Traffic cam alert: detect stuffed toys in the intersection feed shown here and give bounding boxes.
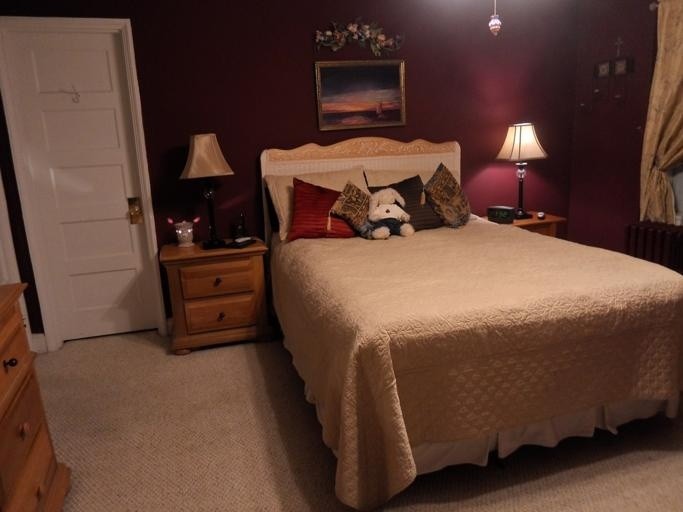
[365,186,414,239]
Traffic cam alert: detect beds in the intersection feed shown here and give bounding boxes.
[260,136,683,512]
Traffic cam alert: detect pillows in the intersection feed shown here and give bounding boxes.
[424,162,471,228]
[368,174,445,232]
[263,166,372,241]
[286,178,354,243]
[362,169,458,189]
[328,180,372,231]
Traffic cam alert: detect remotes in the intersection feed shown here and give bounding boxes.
[234,236,251,243]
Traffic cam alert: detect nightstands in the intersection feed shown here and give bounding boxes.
[480,210,566,239]
[158,237,268,355]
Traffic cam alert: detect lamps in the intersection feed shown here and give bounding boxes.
[495,122,549,219]
[178,133,234,249]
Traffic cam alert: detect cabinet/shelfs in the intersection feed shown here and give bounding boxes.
[0,281,71,512]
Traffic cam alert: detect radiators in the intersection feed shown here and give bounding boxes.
[624,222,682,273]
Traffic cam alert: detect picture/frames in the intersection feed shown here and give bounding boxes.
[314,58,405,131]
